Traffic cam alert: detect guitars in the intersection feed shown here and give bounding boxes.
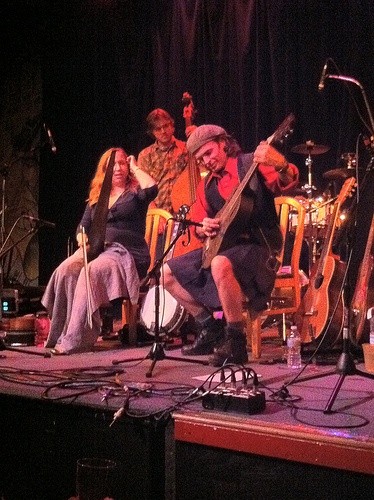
[290,174,359,352]
[199,112,299,270]
[347,212,374,351]
[140,204,190,339]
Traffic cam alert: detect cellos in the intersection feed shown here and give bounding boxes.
[167,90,213,259]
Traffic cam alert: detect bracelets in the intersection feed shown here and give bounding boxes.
[275,162,289,175]
[133,167,140,174]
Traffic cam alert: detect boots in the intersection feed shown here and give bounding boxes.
[98,306,118,340]
[181,319,225,355]
[209,330,248,365]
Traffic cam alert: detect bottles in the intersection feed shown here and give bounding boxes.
[287,326,301,370]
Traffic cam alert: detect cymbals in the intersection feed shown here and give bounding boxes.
[321,168,362,180]
[290,143,330,155]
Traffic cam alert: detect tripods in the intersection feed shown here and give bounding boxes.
[112,227,210,377]
[287,156,374,414]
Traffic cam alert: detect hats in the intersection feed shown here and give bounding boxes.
[187,123,225,153]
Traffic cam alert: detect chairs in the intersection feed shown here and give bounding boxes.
[241,196,305,360]
[112,209,176,344]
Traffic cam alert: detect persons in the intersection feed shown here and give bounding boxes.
[159,125,299,366]
[138,109,207,343]
[41,148,158,354]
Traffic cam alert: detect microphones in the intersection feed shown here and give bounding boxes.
[319,63,327,90]
[47,128,56,151]
[171,217,203,227]
[23,215,56,227]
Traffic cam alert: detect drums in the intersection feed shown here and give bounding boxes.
[291,201,343,240]
